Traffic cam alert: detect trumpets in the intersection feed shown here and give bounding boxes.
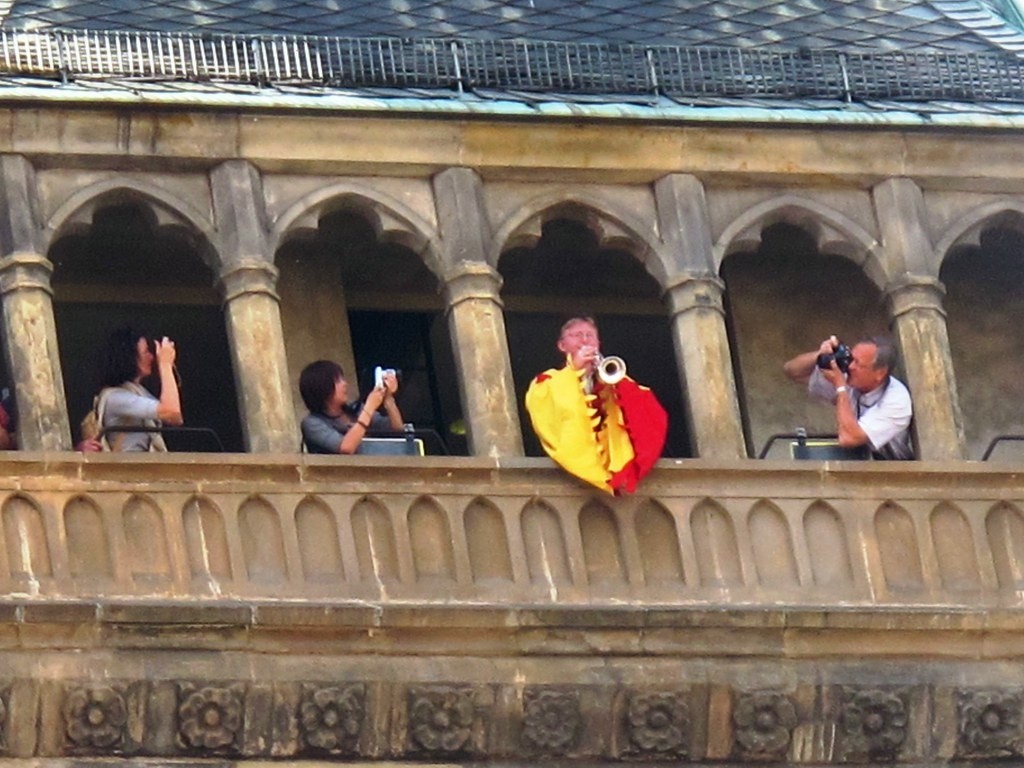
[591,351,627,385]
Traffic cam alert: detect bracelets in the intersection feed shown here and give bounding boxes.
[834,385,850,395]
[355,415,373,435]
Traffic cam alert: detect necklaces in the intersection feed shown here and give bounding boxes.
[321,409,344,419]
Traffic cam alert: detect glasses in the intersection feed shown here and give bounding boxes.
[560,331,599,343]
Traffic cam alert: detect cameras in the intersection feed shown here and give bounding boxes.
[374,367,396,389]
[817,342,852,374]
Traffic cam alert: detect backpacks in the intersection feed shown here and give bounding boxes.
[76,389,134,453]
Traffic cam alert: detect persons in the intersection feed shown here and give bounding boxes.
[783,335,915,461]
[95,325,184,454]
[298,358,404,455]
[525,316,670,498]
[67,405,104,454]
